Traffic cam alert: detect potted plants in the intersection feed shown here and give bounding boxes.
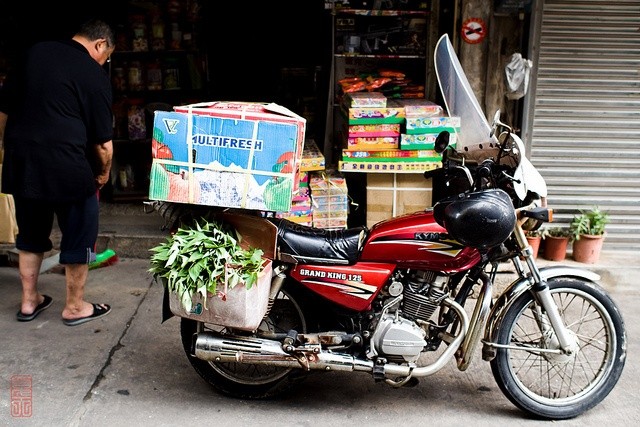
[521,230,542,257]
[571,206,608,264]
[543,224,572,259]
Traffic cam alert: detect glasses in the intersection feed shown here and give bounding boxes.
[105,52,112,62]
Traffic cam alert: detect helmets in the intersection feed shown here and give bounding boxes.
[432,190,516,250]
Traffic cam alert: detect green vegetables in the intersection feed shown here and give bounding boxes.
[145,217,267,315]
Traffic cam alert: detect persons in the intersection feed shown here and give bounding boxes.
[1,17,117,327]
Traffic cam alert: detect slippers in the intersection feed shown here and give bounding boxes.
[65,301,111,326]
[15,294,53,321]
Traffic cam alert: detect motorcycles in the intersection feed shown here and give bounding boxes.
[143,32,629,421]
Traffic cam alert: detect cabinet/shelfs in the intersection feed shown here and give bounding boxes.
[331,1,431,108]
[105,1,200,199]
